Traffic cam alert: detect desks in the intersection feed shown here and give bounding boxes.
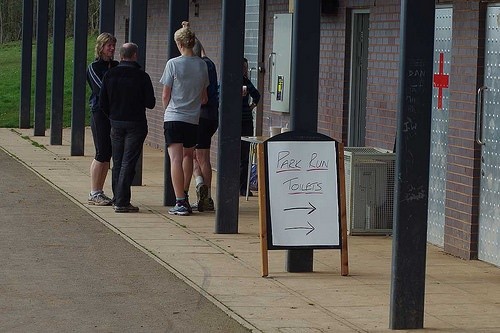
[241,135,270,201]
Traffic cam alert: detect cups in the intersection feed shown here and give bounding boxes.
[243,85,247,96]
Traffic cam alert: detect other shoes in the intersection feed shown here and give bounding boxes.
[241,190,252,196]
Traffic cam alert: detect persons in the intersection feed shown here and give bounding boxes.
[159,28,210,215]
[182,21,219,211]
[99,42,155,212]
[240,58,261,196]
[87,33,119,204]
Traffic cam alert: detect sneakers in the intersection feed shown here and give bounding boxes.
[196,183,209,212]
[168,204,189,214]
[192,198,214,210]
[115,204,139,212]
[88,191,112,205]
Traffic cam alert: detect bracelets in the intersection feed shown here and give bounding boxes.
[252,102,256,106]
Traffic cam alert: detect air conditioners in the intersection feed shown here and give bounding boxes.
[344,158,388,236]
